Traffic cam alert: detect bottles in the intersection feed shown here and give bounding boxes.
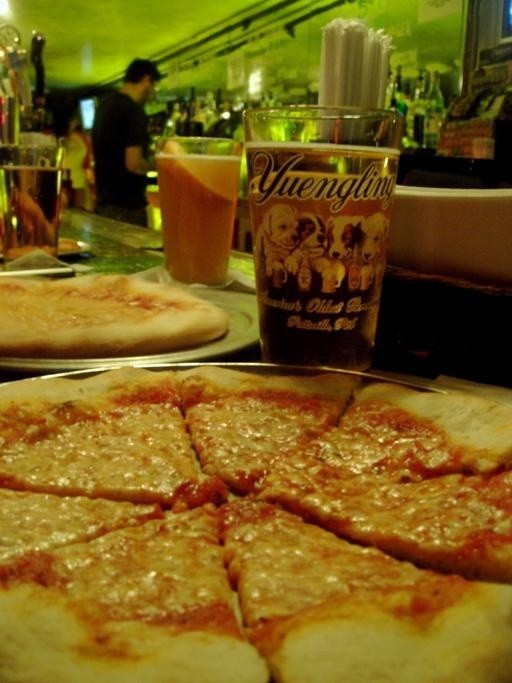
[392,61,445,147]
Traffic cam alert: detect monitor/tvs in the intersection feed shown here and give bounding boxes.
[78,96,98,133]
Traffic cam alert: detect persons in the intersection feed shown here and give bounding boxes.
[91,58,160,228]
[59,110,87,208]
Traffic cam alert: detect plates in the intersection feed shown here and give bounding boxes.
[0,361,453,402]
[4,271,258,366]
[0,236,96,260]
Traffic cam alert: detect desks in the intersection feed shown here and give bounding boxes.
[1,208,512,384]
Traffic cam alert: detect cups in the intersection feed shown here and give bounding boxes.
[0,139,64,262]
[155,134,244,284]
[242,103,404,366]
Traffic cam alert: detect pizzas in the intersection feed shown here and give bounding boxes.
[0,275,227,358]
[0,366,511,682]
